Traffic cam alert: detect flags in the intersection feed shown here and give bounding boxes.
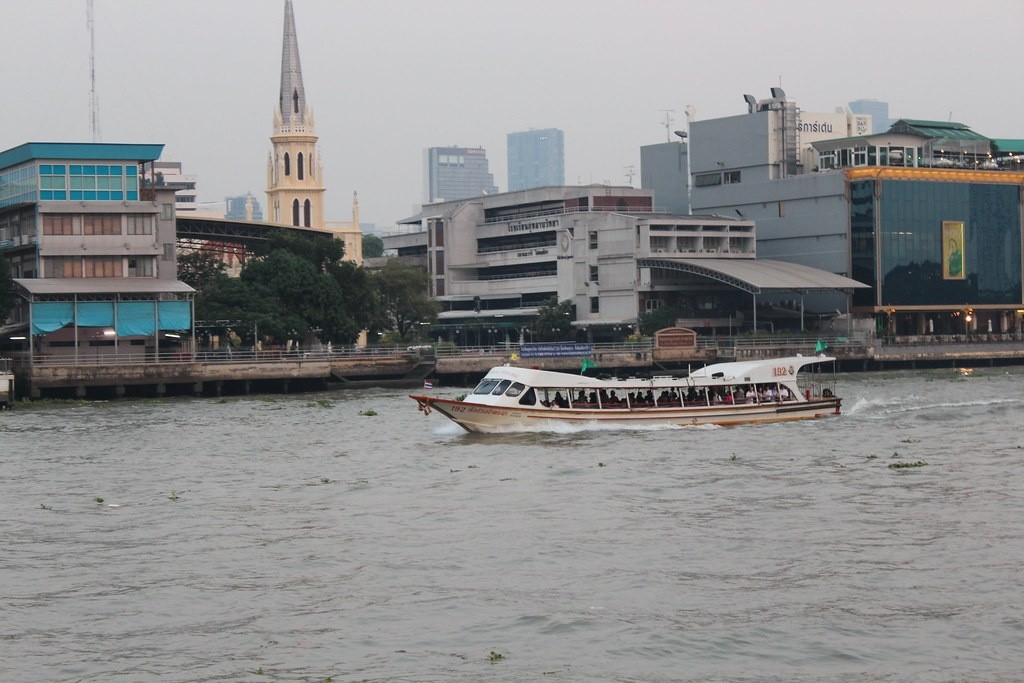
[424,379,433,389]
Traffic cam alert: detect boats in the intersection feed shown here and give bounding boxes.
[409,354,842,434]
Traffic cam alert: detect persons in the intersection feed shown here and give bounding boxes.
[578,387,788,403]
[551,392,565,407]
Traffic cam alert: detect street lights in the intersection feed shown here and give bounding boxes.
[965,303,973,344]
[887,303,896,345]
